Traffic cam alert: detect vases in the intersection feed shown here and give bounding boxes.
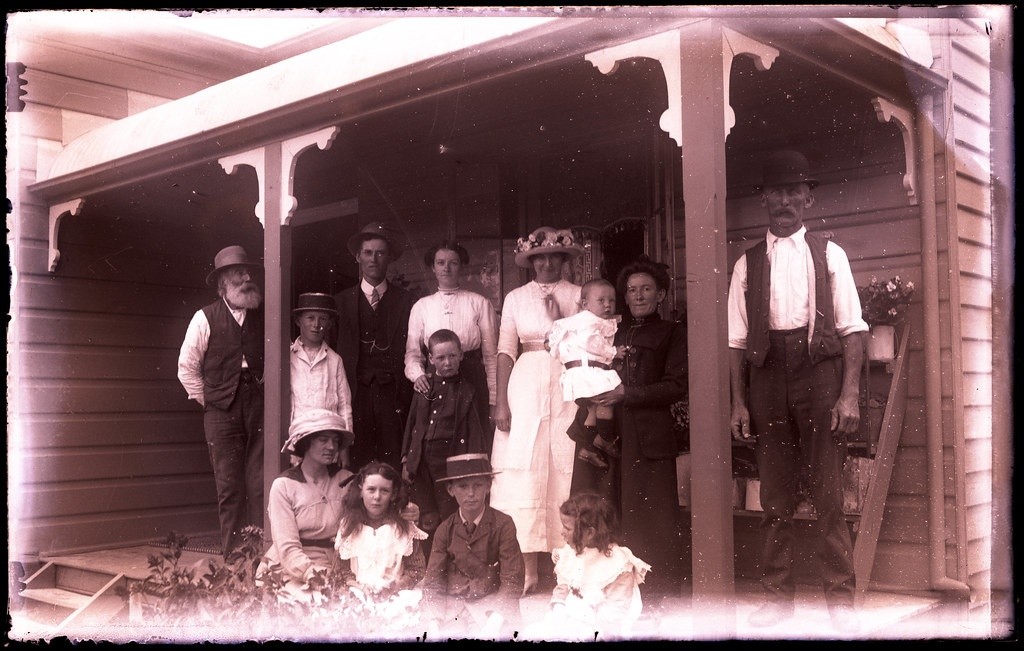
[867,326,898,364]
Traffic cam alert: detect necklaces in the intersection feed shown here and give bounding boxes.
[434,290,458,309]
[535,280,557,298]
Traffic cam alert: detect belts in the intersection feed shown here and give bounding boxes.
[301,537,336,549]
[565,359,609,370]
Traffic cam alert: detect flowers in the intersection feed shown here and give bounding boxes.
[514,229,574,255]
[857,274,916,338]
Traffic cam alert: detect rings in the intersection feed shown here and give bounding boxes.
[600,399,607,404]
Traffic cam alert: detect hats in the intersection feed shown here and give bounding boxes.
[280,410,354,458]
[291,293,338,318]
[346,222,404,263]
[435,454,502,482]
[514,227,587,268]
[751,150,819,190]
[205,246,263,288]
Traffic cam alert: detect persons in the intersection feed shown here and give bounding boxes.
[417,450,525,631]
[728,150,870,608]
[548,277,631,471]
[254,408,421,600]
[399,329,489,569]
[405,240,496,456]
[570,254,689,604]
[289,291,356,475]
[177,243,264,557]
[546,492,652,630]
[333,460,430,616]
[489,225,590,593]
[322,221,420,478]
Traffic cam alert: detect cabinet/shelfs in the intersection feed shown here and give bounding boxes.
[733,323,911,605]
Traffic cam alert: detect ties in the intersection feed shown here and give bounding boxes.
[371,288,381,311]
[466,521,476,537]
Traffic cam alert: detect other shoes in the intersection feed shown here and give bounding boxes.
[578,448,609,469]
[594,435,622,459]
